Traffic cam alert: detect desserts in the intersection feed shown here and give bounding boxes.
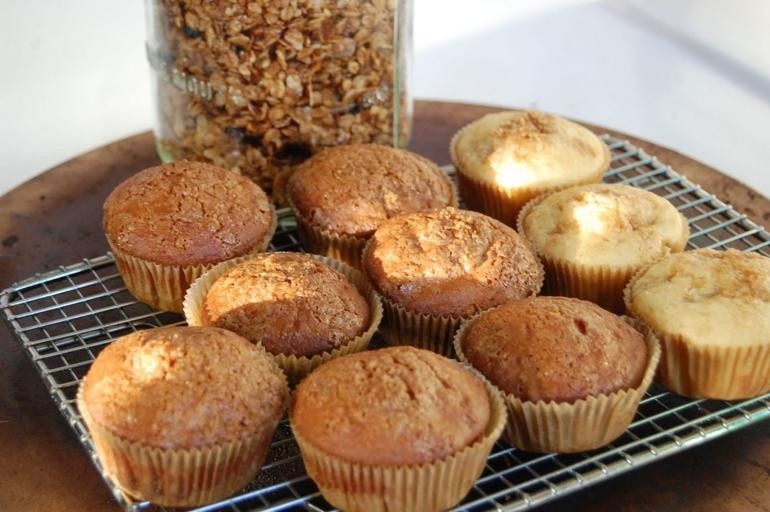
[76,109,769,511]
